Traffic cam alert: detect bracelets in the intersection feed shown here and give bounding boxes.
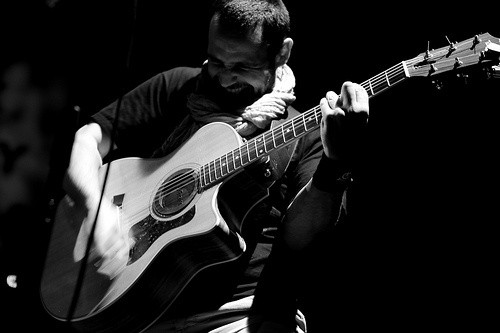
[312,152,356,193]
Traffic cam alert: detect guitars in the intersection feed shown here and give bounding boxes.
[40,32,500,333]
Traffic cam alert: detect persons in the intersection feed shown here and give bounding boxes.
[63,0,371,333]
